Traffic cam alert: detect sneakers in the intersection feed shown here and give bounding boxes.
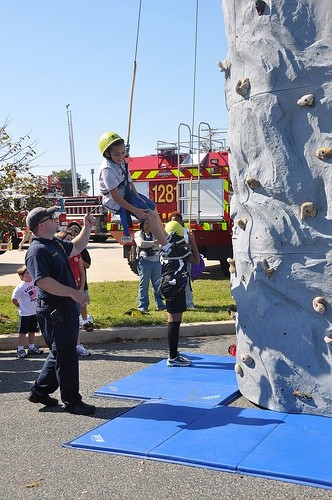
[17,350,30,357]
[166,355,191,366]
[28,347,44,354]
[76,345,92,356]
[166,233,184,243]
[161,243,190,260]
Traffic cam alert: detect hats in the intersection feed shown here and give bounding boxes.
[26,206,57,230]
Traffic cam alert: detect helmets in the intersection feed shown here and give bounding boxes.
[98,132,124,158]
[165,221,184,235]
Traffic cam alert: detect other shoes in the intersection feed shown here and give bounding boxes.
[84,323,94,331]
[65,400,96,413]
[26,392,59,406]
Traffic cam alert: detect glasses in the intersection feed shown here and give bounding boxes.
[36,216,54,226]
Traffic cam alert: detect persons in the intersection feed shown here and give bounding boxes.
[25,206,95,415]
[159,221,200,367]
[12,265,43,358]
[98,131,191,260]
[18,214,32,251]
[67,227,94,332]
[169,211,195,308]
[133,220,166,313]
[58,231,92,357]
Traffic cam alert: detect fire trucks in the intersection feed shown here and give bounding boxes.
[104,122,235,279]
[0,103,110,254]
